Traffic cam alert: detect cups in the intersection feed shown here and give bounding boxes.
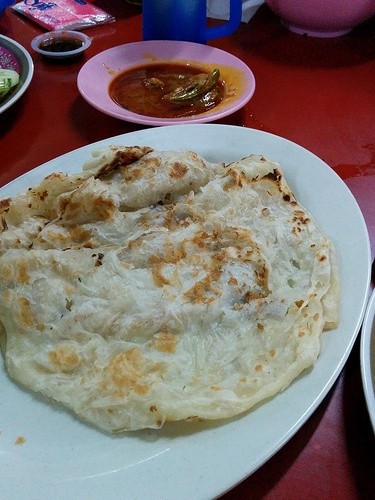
[142,0,242,46]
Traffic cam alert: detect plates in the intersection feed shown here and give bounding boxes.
[77,39,257,124]
[1,35,34,114]
[0,124,373,500]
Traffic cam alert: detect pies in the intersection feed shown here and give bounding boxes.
[0,144,340,435]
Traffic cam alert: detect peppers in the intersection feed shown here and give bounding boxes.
[170,68,220,102]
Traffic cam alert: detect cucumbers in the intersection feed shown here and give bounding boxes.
[0,69,19,98]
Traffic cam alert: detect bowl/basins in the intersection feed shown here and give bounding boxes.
[265,0,375,39]
[30,30,91,60]
